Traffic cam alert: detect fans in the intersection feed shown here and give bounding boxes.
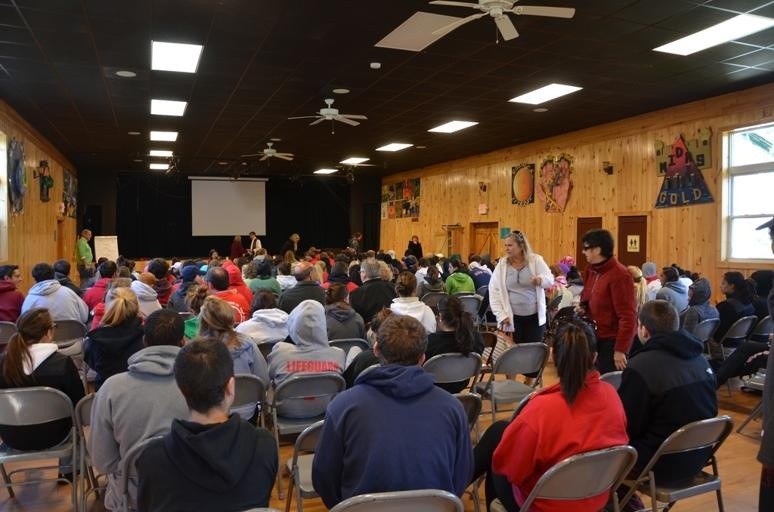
[375,0,576,52]
[288,98,368,135]
[241,143,294,168]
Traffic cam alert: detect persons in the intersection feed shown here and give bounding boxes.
[266,300,346,419]
[419,266,446,300]
[580,230,636,376]
[656,267,689,315]
[91,310,192,512]
[54,261,85,297]
[1,307,84,485]
[756,217,774,512]
[342,309,393,389]
[565,266,585,296]
[360,250,395,260]
[715,341,770,390]
[683,278,719,332]
[470,256,495,287]
[422,297,484,395]
[312,314,474,509]
[391,270,437,338]
[627,266,647,313]
[472,320,629,512]
[197,296,269,426]
[22,264,88,323]
[642,261,662,300]
[234,289,296,362]
[446,260,475,296]
[135,339,278,512]
[619,300,719,512]
[396,255,446,271]
[750,270,774,320]
[84,288,145,391]
[323,283,367,340]
[715,271,756,348]
[322,232,363,290]
[405,235,423,258]
[0,265,25,353]
[489,230,554,384]
[352,260,397,310]
[76,230,325,313]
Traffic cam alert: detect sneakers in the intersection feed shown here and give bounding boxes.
[57,473,77,485]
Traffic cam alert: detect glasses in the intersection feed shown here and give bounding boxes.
[50,322,58,330]
[583,244,595,251]
[556,315,598,331]
[513,229,523,240]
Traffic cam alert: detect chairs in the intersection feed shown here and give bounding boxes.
[490,445,638,512]
[423,353,482,394]
[736,374,765,434]
[421,291,448,318]
[0,387,78,512]
[121,436,165,512]
[454,393,481,431]
[693,318,721,362]
[453,292,475,297]
[470,390,537,501]
[329,489,466,512]
[621,416,735,512]
[480,304,497,334]
[267,373,346,500]
[53,319,89,396]
[599,371,623,391]
[459,295,483,332]
[329,338,369,370]
[543,306,576,341]
[710,316,758,397]
[481,333,497,380]
[748,315,774,343]
[285,419,325,512]
[0,321,19,344]
[75,393,107,512]
[230,374,274,497]
[472,342,550,444]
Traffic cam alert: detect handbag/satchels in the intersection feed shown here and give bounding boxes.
[481,318,515,370]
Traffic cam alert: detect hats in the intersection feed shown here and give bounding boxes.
[182,265,200,278]
[756,218,774,231]
